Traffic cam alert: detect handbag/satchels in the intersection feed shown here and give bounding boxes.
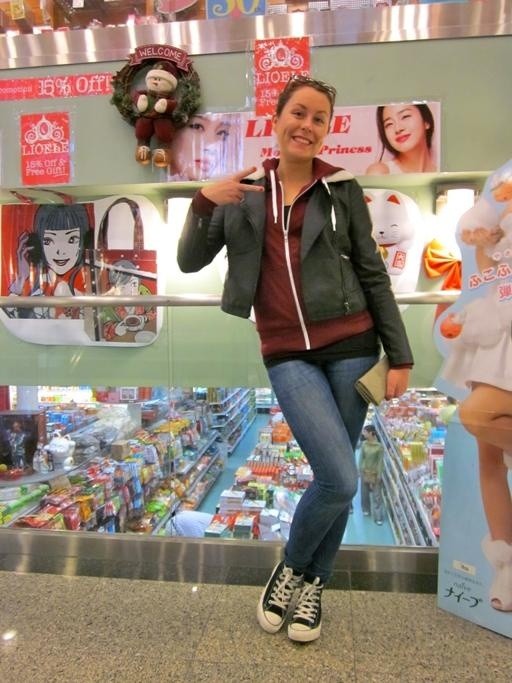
[1,189,96,322]
[84,199,158,345]
[355,351,393,408]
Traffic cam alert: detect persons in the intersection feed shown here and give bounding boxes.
[441,167,512,612]
[166,113,232,180]
[6,422,29,468]
[175,72,416,643]
[166,509,213,539]
[349,499,354,514]
[365,103,438,175]
[358,423,386,526]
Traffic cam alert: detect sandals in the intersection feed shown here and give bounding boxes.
[480,535,511,612]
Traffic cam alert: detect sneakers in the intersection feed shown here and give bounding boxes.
[256,559,303,636]
[287,581,325,643]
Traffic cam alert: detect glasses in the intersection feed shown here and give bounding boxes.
[285,73,338,108]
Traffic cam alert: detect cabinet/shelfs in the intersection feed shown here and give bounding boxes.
[0,386,458,547]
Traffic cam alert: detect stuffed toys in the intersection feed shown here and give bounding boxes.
[361,188,415,277]
[109,44,201,167]
